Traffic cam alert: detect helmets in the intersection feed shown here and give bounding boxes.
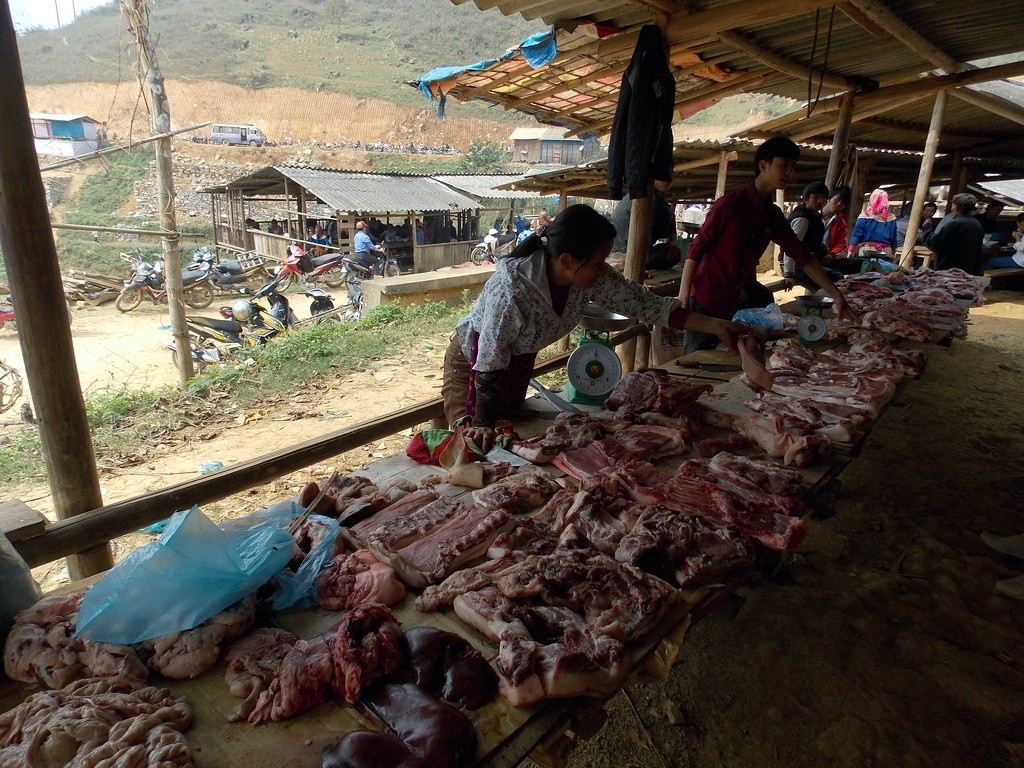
[232,300,252,322]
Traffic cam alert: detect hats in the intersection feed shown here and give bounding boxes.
[356,221,368,229]
[950,193,976,206]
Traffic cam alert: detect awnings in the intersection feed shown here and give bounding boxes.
[404,30,764,140]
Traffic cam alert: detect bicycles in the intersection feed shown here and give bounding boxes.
[345,280,362,308]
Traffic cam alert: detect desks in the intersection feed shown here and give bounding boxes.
[377,242,412,256]
[894,245,1011,269]
[7,269,986,768]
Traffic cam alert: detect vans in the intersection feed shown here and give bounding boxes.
[211,123,267,147]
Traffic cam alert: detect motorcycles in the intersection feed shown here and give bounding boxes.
[182,239,273,297]
[387,142,454,155]
[237,273,301,329]
[264,138,292,147]
[0,295,72,331]
[342,244,400,290]
[116,247,221,313]
[312,138,385,151]
[159,298,285,376]
[471,228,517,266]
[297,287,341,322]
[272,233,345,293]
[187,135,211,144]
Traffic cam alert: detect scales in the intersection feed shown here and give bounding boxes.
[794,296,836,347]
[860,249,887,276]
[564,303,631,406]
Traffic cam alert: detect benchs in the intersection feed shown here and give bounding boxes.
[984,267,1024,285]
[387,254,413,259]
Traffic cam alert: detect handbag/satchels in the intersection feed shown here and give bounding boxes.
[731,302,783,328]
[71,499,339,645]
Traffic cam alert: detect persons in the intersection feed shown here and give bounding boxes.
[90,230,99,242]
[113,133,117,140]
[448,220,456,237]
[922,192,985,270]
[677,136,857,356]
[973,201,1003,233]
[608,180,677,253]
[441,204,748,454]
[896,200,937,247]
[986,212,1024,269]
[307,216,349,246]
[268,219,294,234]
[506,224,513,234]
[539,206,553,225]
[778,181,866,293]
[246,218,261,230]
[354,221,383,278]
[370,217,433,245]
[513,213,527,234]
[847,189,897,263]
[97,129,107,140]
[493,216,504,237]
[516,222,534,246]
